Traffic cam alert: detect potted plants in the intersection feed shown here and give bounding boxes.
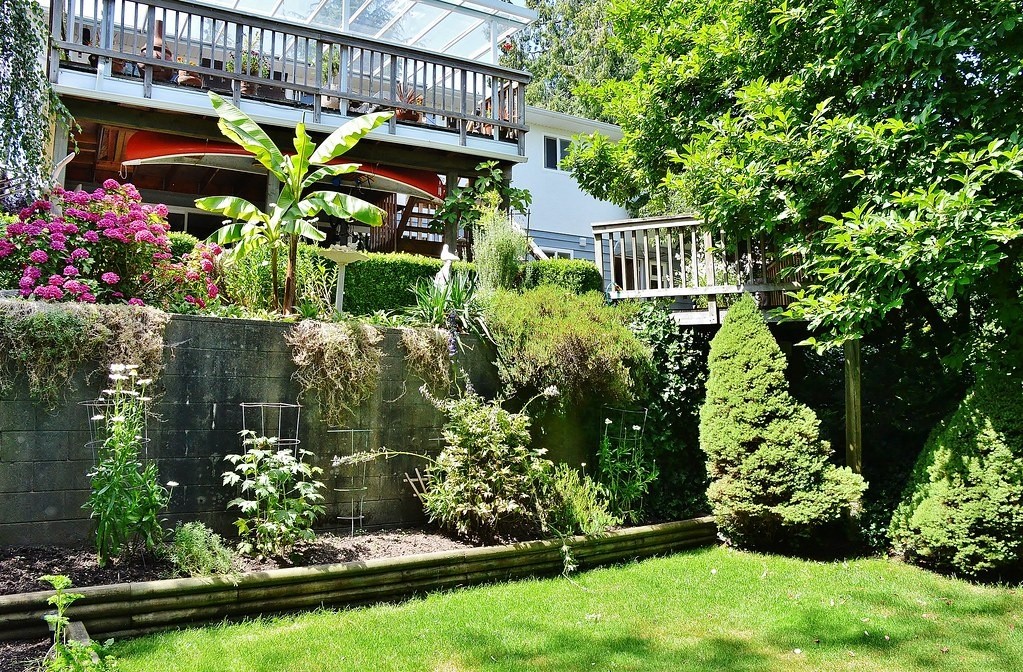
[384,83,423,123]
[485,105,510,138]
[88,34,126,71]
[308,41,340,109]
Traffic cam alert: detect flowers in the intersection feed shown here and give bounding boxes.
[224,47,270,79]
[177,57,196,66]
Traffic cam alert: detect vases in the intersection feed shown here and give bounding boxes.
[231,73,259,96]
[177,71,202,87]
